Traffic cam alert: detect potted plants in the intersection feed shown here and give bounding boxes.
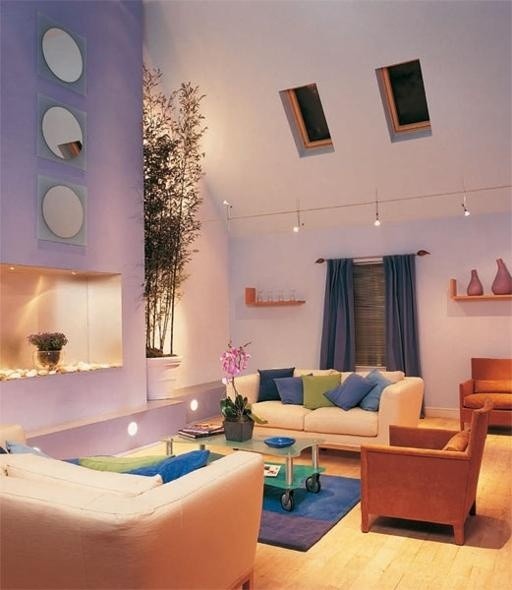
[141,65,208,400]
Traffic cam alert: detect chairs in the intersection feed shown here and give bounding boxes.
[359,396,495,545]
[458,357,511,431]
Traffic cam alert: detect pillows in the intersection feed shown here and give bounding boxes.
[257,368,395,412]
[0,441,210,486]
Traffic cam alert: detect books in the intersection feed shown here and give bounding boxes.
[176,424,225,442]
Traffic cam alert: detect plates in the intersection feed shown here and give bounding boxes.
[264,437,295,448]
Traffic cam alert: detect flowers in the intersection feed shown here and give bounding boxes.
[27,330,68,366]
[219,338,268,425]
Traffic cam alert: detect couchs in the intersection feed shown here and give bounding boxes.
[225,370,425,452]
[1,424,265,590]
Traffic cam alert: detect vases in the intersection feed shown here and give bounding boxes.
[222,420,256,443]
[33,350,66,372]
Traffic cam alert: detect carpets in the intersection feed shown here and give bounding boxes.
[209,450,360,553]
